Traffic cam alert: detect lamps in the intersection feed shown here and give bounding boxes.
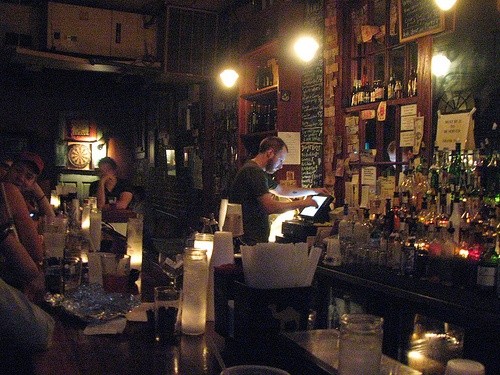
[97,139,108,149]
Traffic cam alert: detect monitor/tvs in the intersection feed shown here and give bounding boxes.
[299,193,334,221]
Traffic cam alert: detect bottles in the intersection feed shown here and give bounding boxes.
[351,67,417,107]
[338,313,384,375]
[339,143,500,292]
[214,101,238,133]
[72,199,80,221]
[248,65,276,134]
[183,233,214,336]
[127,217,143,273]
[82,196,102,252]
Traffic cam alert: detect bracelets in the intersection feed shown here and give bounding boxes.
[0,222,14,241]
[37,193,46,202]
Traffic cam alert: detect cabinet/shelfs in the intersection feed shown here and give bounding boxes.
[342,0,429,199]
[177,0,306,204]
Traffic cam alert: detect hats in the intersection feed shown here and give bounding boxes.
[18,152,43,175]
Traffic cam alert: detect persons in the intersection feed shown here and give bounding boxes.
[0,151,56,353]
[230,136,318,246]
[89,156,134,210]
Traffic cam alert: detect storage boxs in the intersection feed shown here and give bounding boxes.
[0,0,161,64]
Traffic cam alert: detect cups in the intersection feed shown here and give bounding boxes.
[101,253,131,292]
[62,256,82,292]
[153,285,182,331]
[210,232,235,268]
[223,203,244,236]
